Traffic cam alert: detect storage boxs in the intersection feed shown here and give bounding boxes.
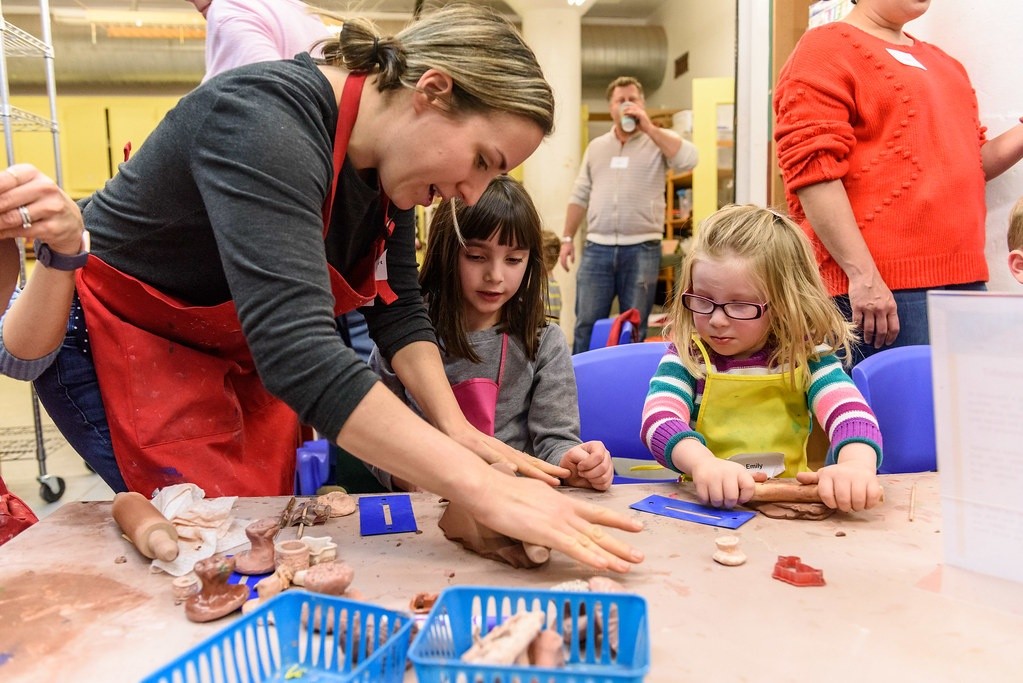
[404,585,649,683]
[142,588,414,682]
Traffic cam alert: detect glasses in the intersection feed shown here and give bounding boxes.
[679,287,775,322]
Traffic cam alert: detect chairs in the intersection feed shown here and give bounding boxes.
[293,316,939,498]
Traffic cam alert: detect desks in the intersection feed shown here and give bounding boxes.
[0,471,1023,680]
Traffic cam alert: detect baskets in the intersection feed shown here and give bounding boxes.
[405,587,650,683]
[140,587,414,683]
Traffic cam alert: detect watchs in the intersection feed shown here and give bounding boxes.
[561,235,574,243]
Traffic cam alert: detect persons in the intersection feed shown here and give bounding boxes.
[0,162,92,381]
[772,0,1022,385]
[33,2,647,577]
[638,202,886,513]
[558,76,701,355]
[360,170,615,493]
[186,0,341,86]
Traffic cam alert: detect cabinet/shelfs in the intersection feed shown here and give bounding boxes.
[0,0,98,504]
[657,168,693,311]
